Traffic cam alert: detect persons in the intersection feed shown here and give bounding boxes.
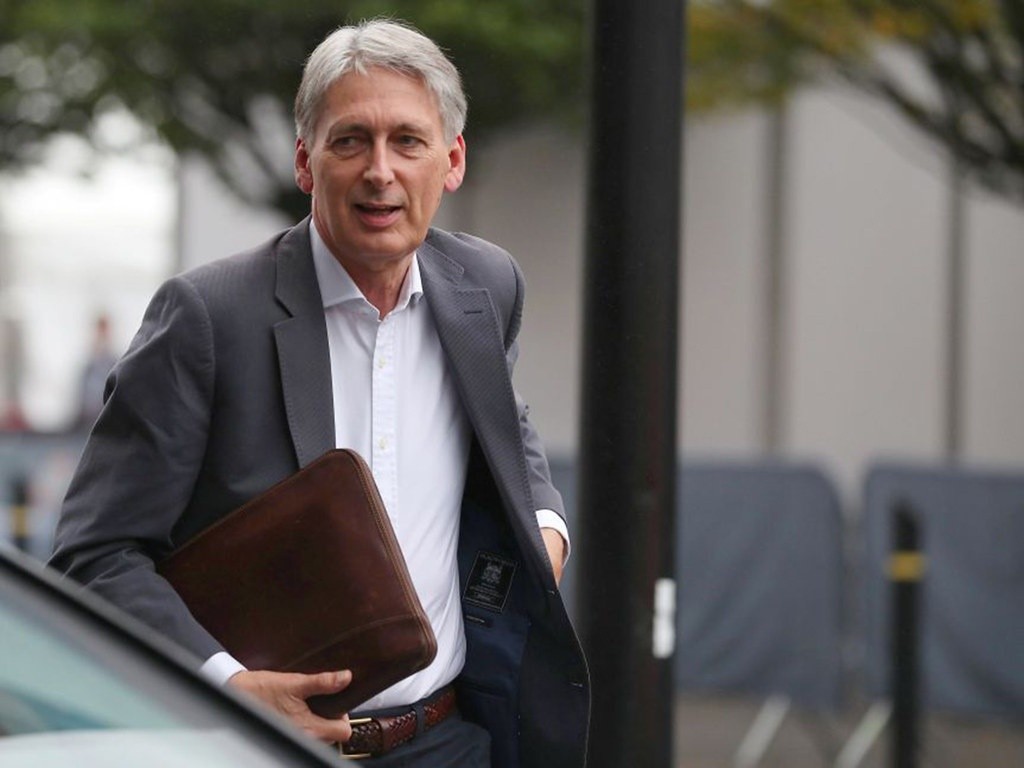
[49,20,591,768]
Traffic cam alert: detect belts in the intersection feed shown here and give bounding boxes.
[332,690,457,762]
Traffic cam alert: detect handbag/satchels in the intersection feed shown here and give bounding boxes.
[151,450,438,721]
